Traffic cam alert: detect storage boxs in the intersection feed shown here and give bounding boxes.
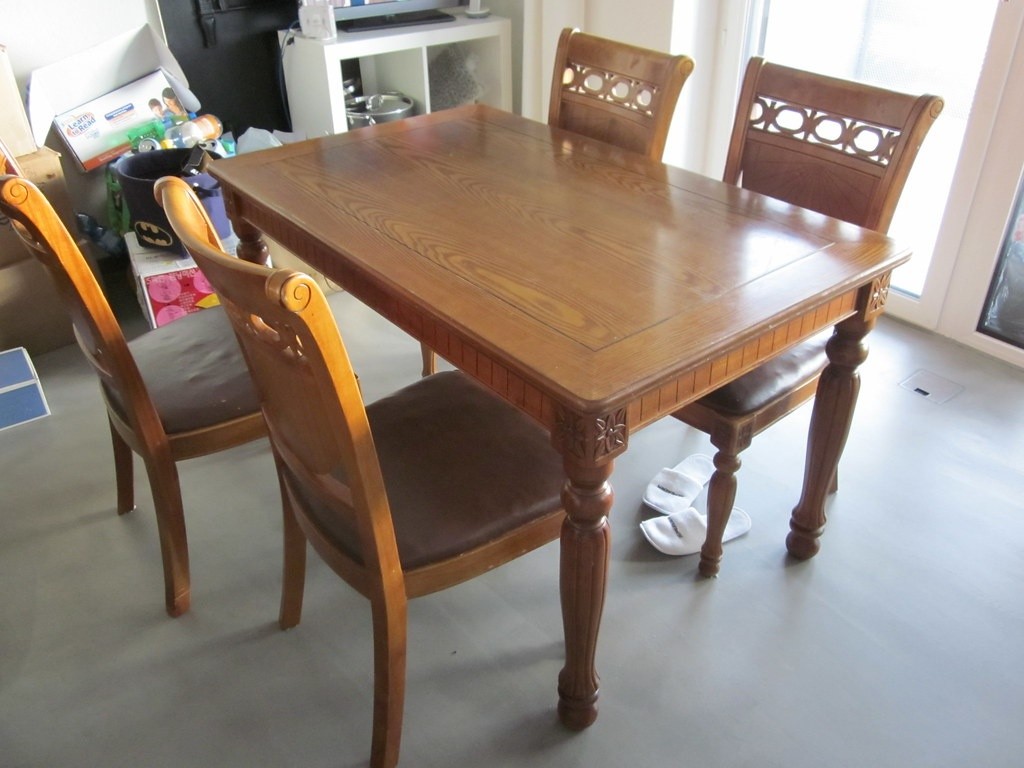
[260,232,345,295]
[0,347,52,433]
[48,69,188,173]
[0,147,78,270]
[125,218,273,329]
[0,238,108,359]
[0,43,36,159]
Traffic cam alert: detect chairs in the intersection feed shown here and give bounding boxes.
[667,58,944,577]
[153,175,570,768]
[0,153,361,617]
[547,26,694,161]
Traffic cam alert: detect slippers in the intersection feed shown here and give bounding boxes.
[641,453,718,515]
[639,507,752,556]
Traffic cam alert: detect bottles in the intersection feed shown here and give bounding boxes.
[160,113,223,149]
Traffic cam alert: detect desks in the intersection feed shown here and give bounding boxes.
[207,103,912,731]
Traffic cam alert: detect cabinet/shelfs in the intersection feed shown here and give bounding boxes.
[278,7,513,137]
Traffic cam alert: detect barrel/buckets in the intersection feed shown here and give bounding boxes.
[115,148,232,259]
[345,90,414,128]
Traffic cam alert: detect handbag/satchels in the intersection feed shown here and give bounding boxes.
[116,148,232,260]
